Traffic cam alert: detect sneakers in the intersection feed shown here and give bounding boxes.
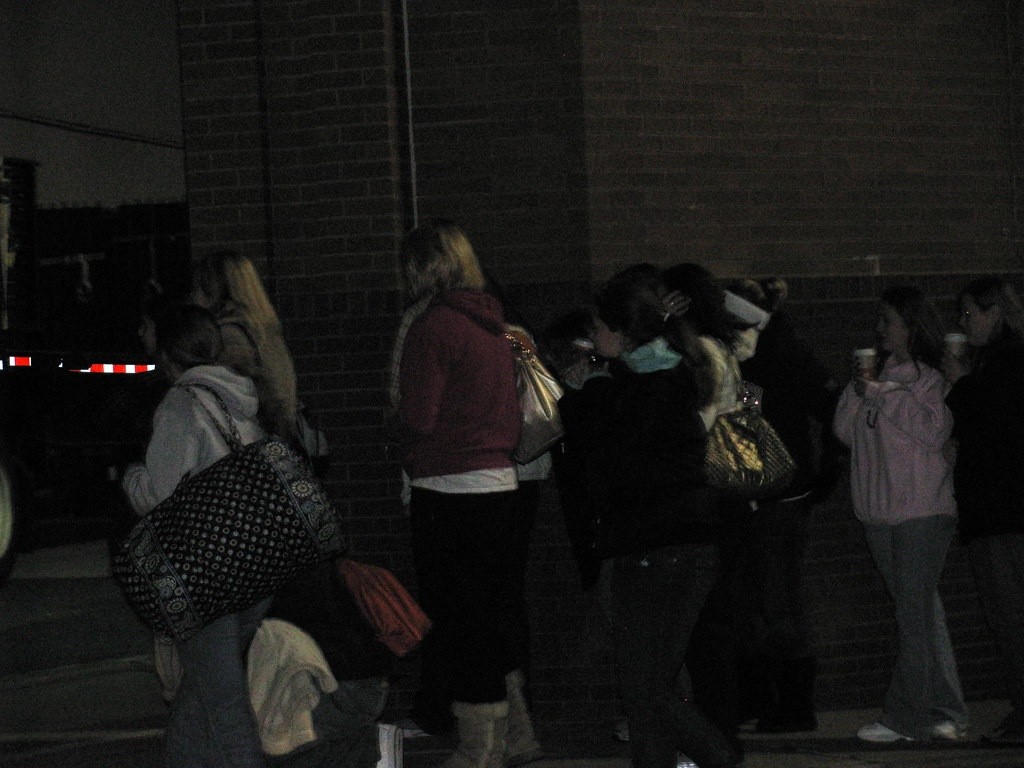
[933,719,970,738]
[857,722,914,742]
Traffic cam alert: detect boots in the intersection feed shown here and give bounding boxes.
[377,721,404,768]
[505,670,545,766]
[438,701,509,767]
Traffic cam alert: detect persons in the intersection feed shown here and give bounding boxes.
[944,275,1024,745]
[384,216,546,768]
[101,244,404,768]
[539,258,831,768]
[831,284,969,742]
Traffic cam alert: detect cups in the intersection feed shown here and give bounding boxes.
[561,340,595,390]
[852,349,876,394]
[942,333,968,366]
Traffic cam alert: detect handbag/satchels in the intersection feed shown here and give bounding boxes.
[114,384,346,645]
[506,333,568,465]
[334,558,432,659]
[703,335,804,498]
